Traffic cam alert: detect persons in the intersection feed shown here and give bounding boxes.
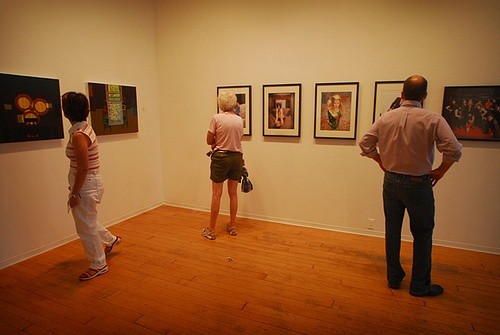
[360,75,463,297]
[63,92,122,281]
[202,91,244,240]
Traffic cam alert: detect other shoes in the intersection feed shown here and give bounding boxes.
[389,281,401,289]
[414,284,444,297]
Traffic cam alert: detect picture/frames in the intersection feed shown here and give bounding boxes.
[314,82,359,140]
[373,81,423,123]
[263,84,301,137]
[216,85,252,135]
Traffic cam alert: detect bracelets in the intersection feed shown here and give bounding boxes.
[69,193,81,199]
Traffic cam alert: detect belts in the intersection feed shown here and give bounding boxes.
[216,150,240,154]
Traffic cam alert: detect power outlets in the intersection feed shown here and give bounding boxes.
[368,218,375,229]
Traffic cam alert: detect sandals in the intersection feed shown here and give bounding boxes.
[225,223,238,236]
[104,236,122,256]
[201,227,216,240]
[79,265,109,280]
